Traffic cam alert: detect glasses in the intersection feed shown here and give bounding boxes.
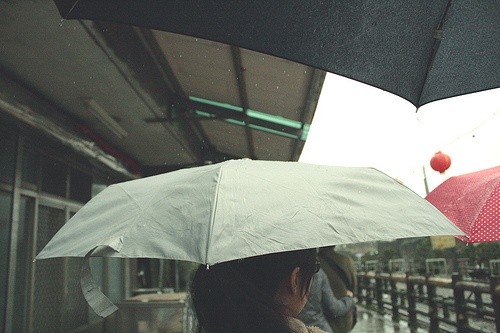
[314,258,321,273]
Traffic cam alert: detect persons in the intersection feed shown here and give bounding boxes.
[189,248,330,333]
[303,266,353,333]
[317,245,357,333]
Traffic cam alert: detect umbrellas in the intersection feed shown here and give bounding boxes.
[424,165,500,247]
[52,0,500,114]
[33,158,470,319]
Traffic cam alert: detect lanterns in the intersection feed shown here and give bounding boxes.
[429,151,451,174]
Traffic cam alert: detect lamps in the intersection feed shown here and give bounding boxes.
[83,97,128,138]
